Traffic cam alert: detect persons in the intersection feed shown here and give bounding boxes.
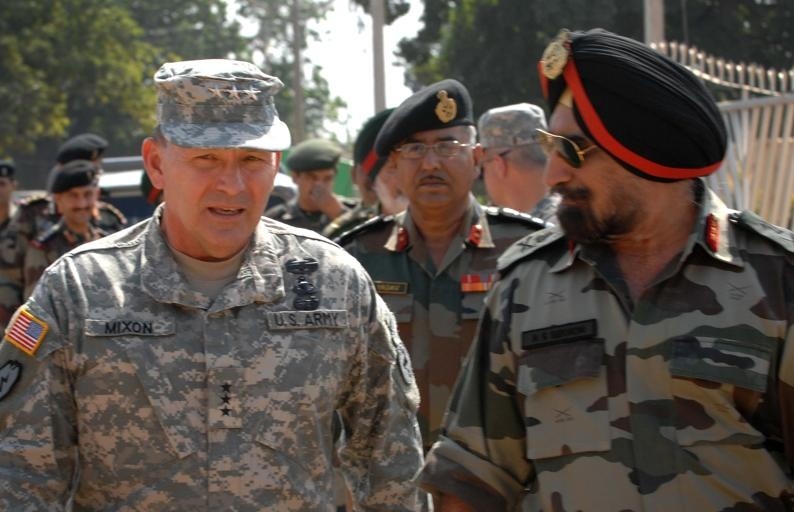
[412,25,792,512]
[265,103,563,242]
[0,134,164,339]
[331,75,560,462]
[0,57,444,512]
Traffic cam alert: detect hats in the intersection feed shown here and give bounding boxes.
[540,28,728,183]
[1,133,107,196]
[153,58,291,152]
[286,79,548,186]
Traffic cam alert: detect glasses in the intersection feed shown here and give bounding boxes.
[534,129,598,166]
